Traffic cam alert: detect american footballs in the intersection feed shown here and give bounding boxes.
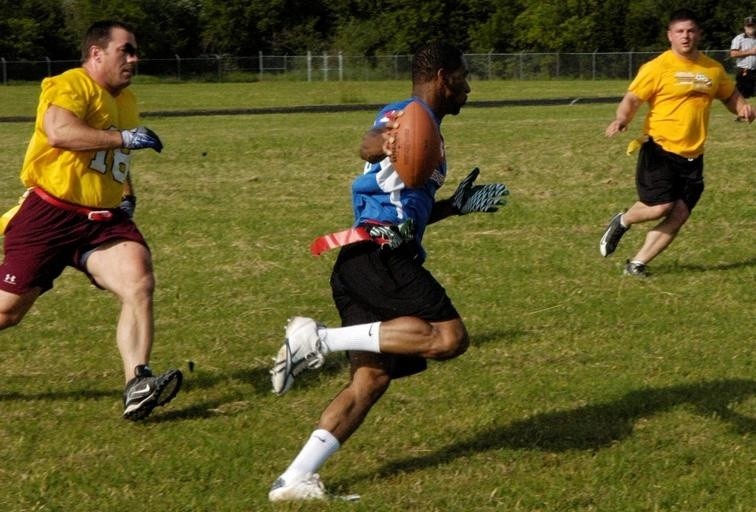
[392,101,441,191]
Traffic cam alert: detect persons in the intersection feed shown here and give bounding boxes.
[268,43,509,500]
[600,11,756,278]
[730,17,756,121]
[0,20,184,422]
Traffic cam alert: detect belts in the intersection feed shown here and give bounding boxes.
[33,186,112,222]
[312,227,404,252]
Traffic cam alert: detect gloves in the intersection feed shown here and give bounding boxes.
[436,167,508,214]
[120,193,136,220]
[122,127,164,150]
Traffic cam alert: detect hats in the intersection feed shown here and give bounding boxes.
[744,15,756,31]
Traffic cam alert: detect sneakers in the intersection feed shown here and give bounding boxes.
[121,366,184,415]
[270,315,323,394]
[624,263,644,278]
[599,212,628,258]
[269,477,357,503]
